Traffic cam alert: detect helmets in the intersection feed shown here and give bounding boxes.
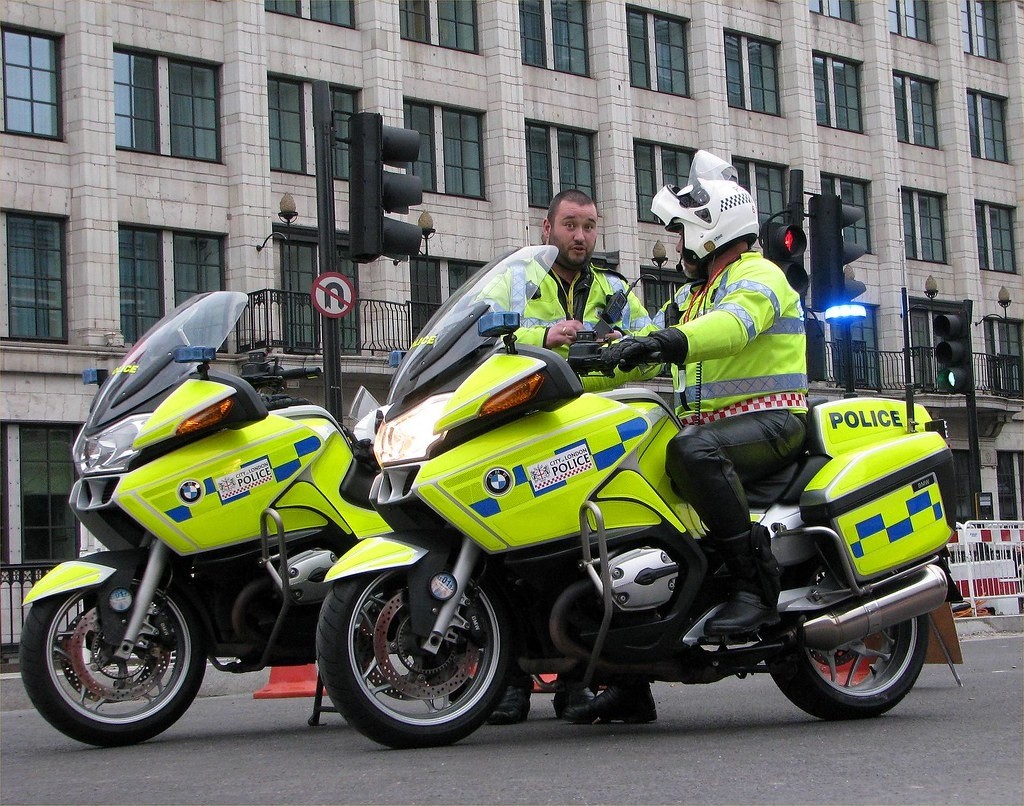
[650,149,760,267]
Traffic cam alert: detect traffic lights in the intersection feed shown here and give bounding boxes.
[766,222,809,299]
[347,110,425,266]
[809,193,865,313]
[926,310,968,395]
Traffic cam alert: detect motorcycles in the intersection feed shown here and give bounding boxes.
[311,244,966,749]
[16,289,396,749]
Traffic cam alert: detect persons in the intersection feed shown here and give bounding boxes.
[558,178,809,724]
[487,190,660,726]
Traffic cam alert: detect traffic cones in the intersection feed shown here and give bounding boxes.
[250,661,326,698]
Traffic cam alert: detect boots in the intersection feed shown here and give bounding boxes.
[703,522,782,637]
[565,681,656,724]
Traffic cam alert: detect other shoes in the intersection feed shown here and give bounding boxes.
[488,684,530,723]
[553,687,598,721]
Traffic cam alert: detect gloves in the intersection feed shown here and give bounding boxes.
[596,333,660,377]
